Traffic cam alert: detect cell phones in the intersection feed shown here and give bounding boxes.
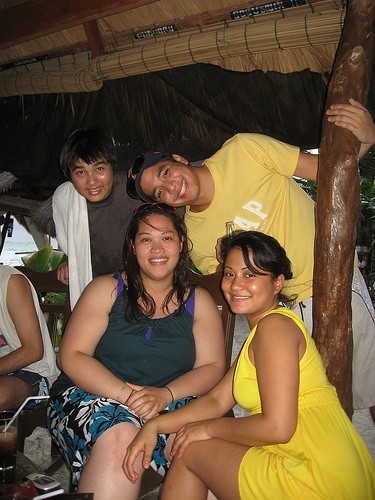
[25,473,62,492]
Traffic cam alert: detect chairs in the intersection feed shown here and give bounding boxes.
[186,271,236,418]
[13,263,72,456]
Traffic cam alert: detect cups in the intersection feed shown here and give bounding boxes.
[0,410,18,472]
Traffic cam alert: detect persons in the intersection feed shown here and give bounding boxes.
[0,266,59,413]
[52,200,227,500]
[113,232,375,500]
[127,98,375,424]
[52,127,184,300]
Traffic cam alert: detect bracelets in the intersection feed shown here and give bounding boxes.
[123,389,134,405]
[164,386,174,403]
